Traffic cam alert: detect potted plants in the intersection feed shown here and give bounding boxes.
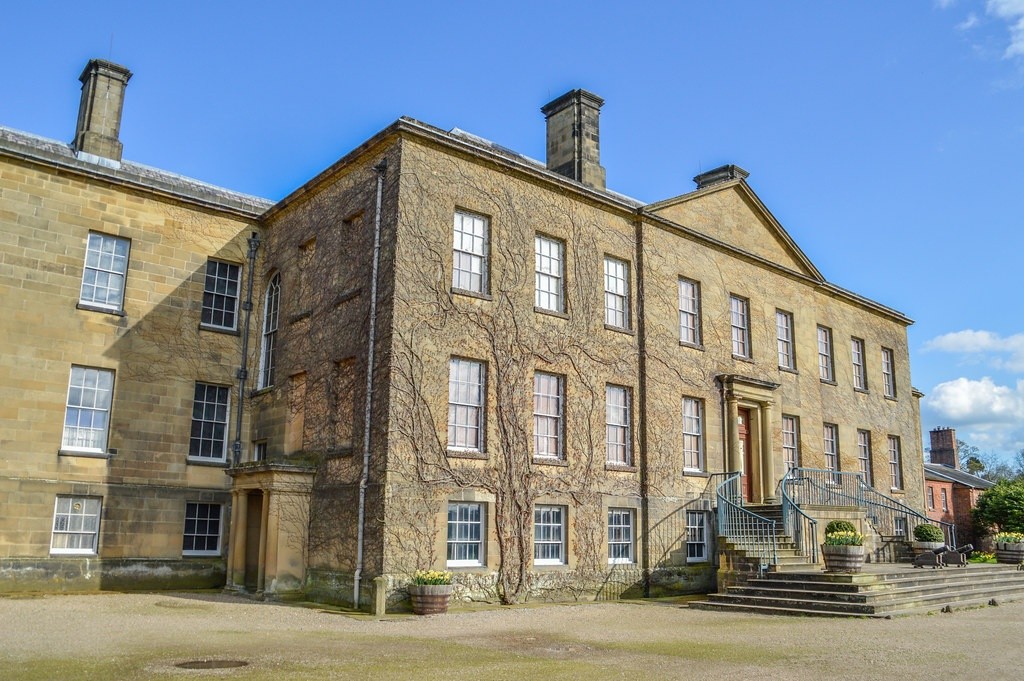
[911,523,945,563]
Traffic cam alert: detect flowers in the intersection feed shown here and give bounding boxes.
[414,568,453,585]
[992,532,1024,543]
[825,531,865,546]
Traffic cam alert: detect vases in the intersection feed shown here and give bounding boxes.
[992,541,1024,564]
[408,584,453,615]
[819,544,865,575]
